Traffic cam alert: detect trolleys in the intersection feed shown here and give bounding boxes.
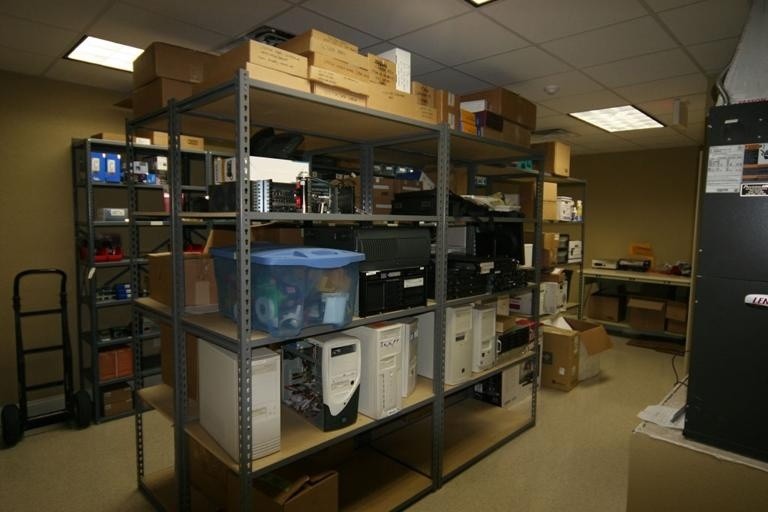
[0,269,93,446]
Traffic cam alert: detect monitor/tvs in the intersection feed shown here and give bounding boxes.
[473,222,526,266]
[447,226,467,253]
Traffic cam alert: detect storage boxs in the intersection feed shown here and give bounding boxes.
[129,30,411,95]
[366,80,438,125]
[459,107,477,135]
[146,134,204,152]
[464,88,537,131]
[540,287,688,392]
[526,231,558,268]
[147,252,219,316]
[532,142,571,176]
[209,245,363,333]
[478,118,532,146]
[91,134,140,144]
[494,179,557,221]
[255,465,340,510]
[433,89,459,127]
[129,78,198,114]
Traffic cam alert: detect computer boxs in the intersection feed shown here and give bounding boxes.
[472,303,497,373]
[197,338,281,463]
[416,303,474,386]
[398,317,419,399]
[347,321,403,421]
[280,331,362,433]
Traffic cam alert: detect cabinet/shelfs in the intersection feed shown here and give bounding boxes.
[70,134,227,428]
[123,72,583,510]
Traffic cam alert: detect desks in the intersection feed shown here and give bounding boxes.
[578,267,690,339]
